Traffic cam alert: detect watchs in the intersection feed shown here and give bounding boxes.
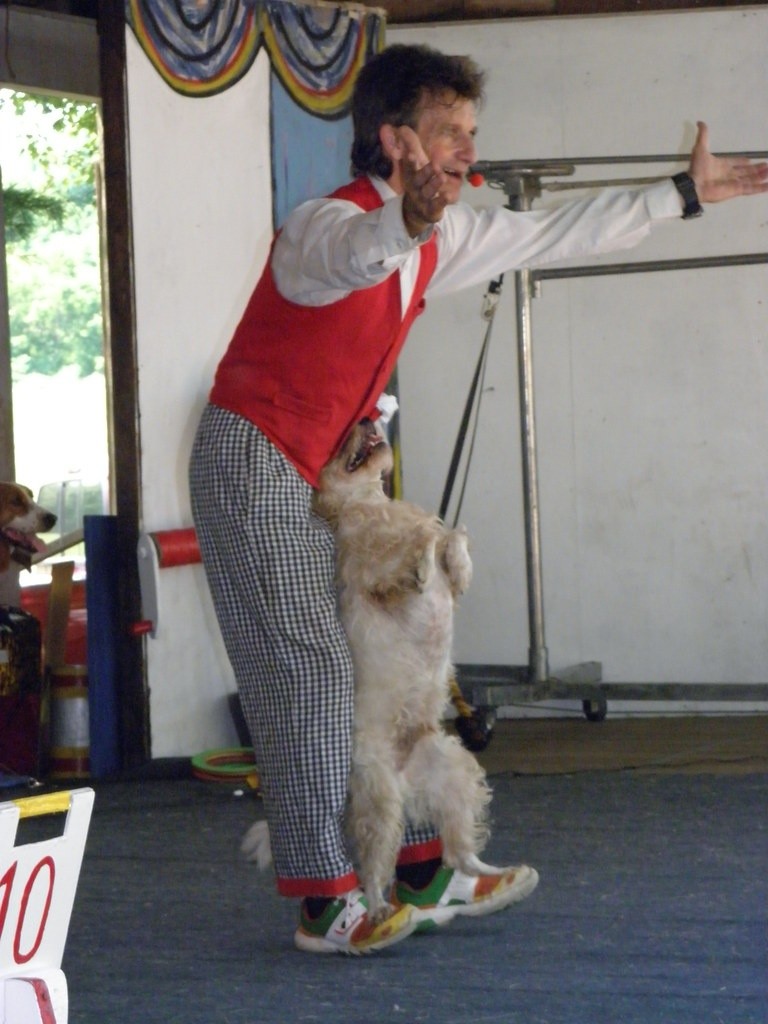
[671,170,704,222]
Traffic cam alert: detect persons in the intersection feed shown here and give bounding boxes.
[189,43,767,955]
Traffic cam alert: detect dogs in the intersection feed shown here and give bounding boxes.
[238,416,521,927]
[1,482,59,571]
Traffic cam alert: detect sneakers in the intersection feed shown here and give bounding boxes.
[294,888,422,956]
[387,855,539,933]
